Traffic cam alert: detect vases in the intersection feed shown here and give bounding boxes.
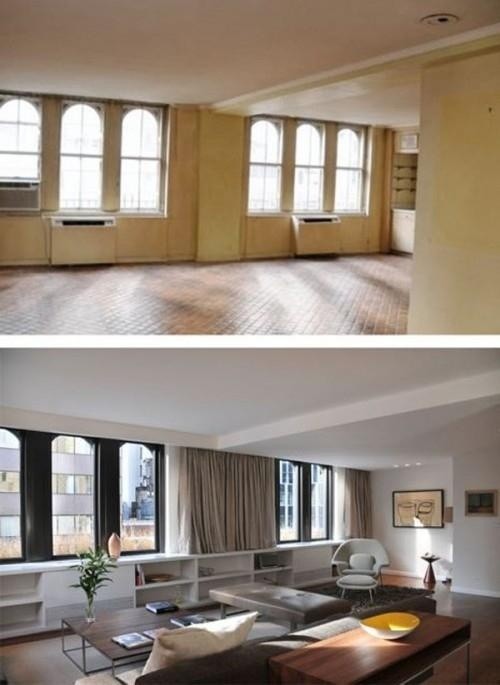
[107,532,121,558]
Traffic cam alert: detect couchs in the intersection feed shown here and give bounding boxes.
[74,595,436,685]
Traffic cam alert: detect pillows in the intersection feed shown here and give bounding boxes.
[348,553,374,569]
[141,611,258,675]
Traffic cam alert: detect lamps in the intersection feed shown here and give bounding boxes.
[444,506,453,522]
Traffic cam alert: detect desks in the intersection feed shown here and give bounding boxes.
[268,609,472,685]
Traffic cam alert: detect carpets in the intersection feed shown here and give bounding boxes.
[226,582,436,630]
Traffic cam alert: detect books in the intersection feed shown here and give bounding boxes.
[137,565,173,585]
[170,613,208,627]
[146,600,179,613]
[144,627,169,639]
[113,632,153,648]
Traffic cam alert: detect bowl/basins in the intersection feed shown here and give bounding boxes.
[358,611,420,640]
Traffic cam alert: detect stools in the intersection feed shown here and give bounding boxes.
[336,573,378,603]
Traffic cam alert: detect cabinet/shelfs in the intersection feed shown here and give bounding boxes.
[61,607,218,677]
[1,539,345,641]
[391,208,415,259]
[393,164,416,192]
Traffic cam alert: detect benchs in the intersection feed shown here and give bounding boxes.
[208,582,352,633]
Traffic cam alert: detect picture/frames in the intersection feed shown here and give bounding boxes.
[392,489,444,529]
[464,489,500,517]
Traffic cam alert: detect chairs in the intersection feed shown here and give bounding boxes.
[332,538,390,589]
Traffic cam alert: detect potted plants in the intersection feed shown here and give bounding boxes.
[68,545,118,622]
